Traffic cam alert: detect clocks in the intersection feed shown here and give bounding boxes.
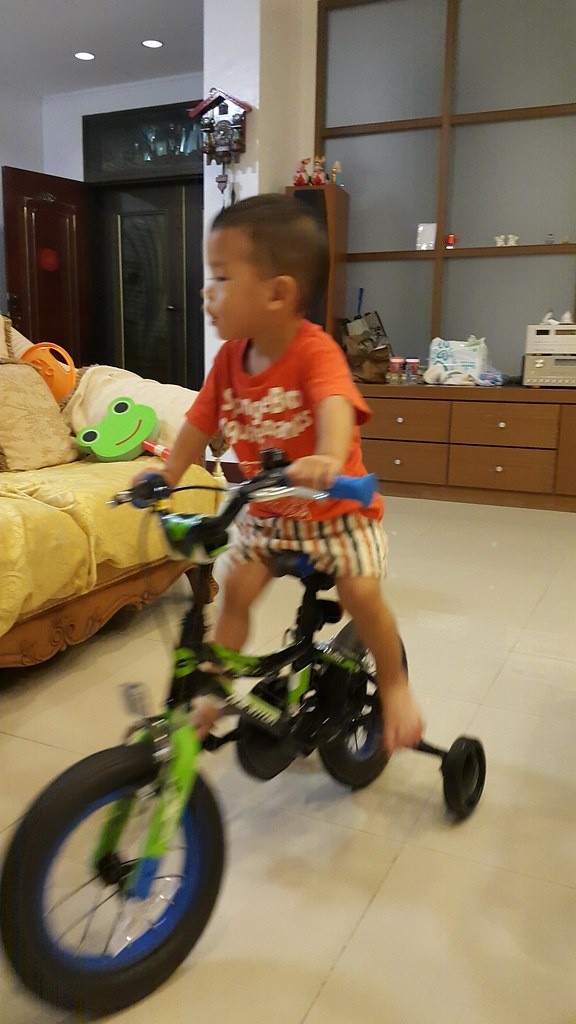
[188,87,253,214]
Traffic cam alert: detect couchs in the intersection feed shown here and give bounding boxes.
[0,315,230,669]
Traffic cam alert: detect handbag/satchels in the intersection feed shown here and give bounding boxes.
[345,310,394,356]
[343,329,392,385]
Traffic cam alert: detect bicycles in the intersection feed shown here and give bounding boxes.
[1,451,487,1018]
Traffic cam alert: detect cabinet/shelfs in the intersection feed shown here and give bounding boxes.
[283,183,352,345]
[357,395,564,493]
[557,403,576,496]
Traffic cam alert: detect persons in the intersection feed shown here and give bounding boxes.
[131,192,426,758]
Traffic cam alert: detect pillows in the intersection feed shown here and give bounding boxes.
[1,360,87,473]
